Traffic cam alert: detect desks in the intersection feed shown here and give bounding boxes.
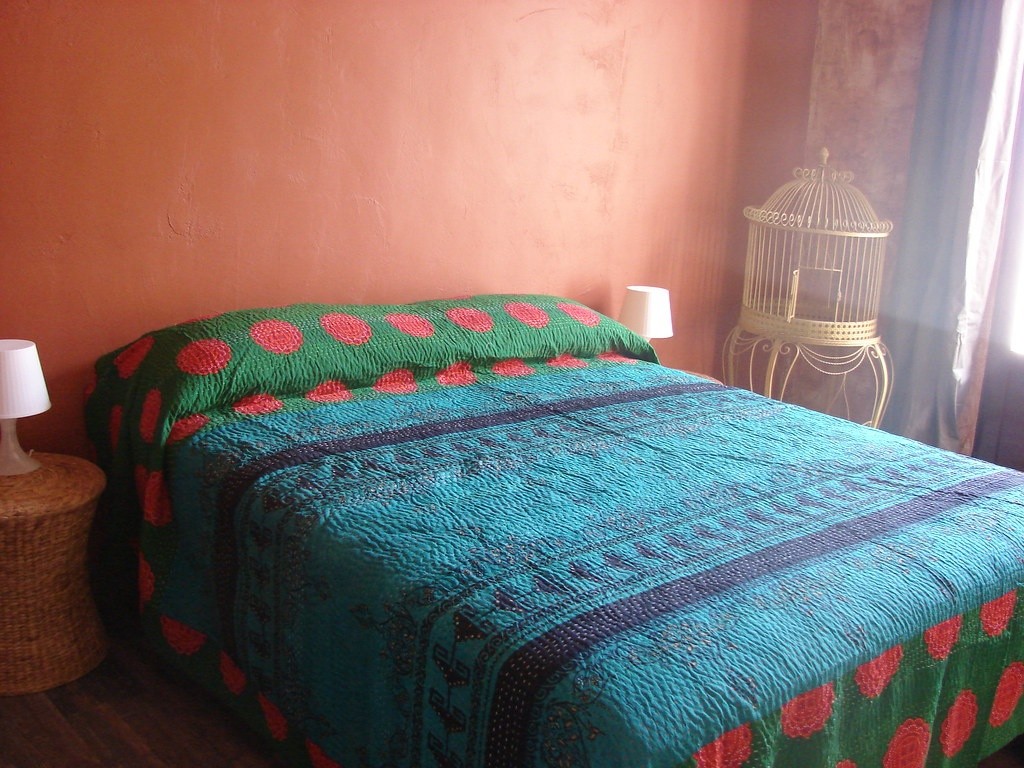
[0,451,107,697]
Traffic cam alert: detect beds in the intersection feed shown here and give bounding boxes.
[86,294,1024,768]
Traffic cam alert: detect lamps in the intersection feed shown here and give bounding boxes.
[0,339,52,478]
[618,286,674,344]
